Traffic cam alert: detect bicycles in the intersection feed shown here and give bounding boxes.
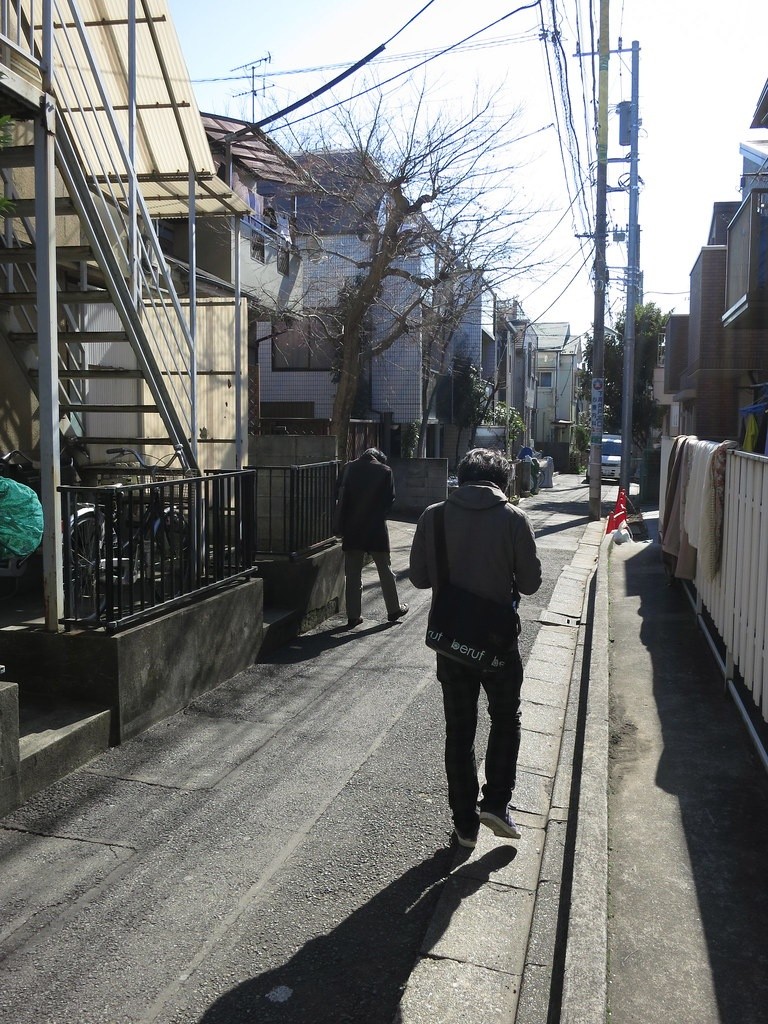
[60,442,192,623]
[0,444,89,606]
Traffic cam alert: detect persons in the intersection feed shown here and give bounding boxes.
[409,447,542,849]
[335,447,409,627]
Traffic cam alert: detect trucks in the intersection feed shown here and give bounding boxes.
[585,435,622,484]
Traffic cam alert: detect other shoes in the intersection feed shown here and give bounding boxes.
[347,615,363,628]
[454,821,479,848]
[479,800,520,839]
[388,603,409,622]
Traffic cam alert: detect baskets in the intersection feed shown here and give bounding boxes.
[9,469,41,495]
[38,455,76,489]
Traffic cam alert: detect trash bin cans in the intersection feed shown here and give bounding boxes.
[522,458,539,495]
[539,457,553,488]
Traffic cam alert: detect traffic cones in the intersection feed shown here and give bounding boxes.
[614,488,627,529]
[605,511,616,535]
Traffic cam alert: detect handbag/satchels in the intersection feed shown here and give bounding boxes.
[333,460,352,538]
[426,581,517,673]
